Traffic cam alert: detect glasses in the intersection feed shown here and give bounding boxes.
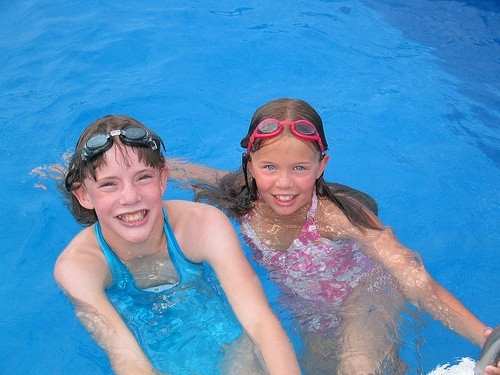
[80,125,167,162]
[247,118,324,155]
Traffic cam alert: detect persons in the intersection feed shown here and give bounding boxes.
[55,115,302,375]
[197,98,500,375]
